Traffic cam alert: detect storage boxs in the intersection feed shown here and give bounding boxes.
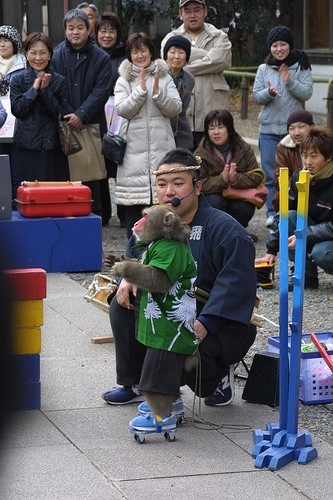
[268,332,333,403]
[16,184,92,217]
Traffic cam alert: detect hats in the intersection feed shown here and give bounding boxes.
[268,25,293,50]
[0,25,22,54]
[179,0,205,8]
[287,109,313,127]
[163,36,190,64]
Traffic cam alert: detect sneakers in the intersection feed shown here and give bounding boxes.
[138,397,184,418]
[102,383,146,406]
[129,409,177,432]
[205,363,234,406]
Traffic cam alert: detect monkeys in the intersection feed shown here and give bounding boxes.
[96,201,200,446]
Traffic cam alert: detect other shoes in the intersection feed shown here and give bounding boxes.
[287,272,318,290]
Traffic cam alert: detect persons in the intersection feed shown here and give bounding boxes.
[114,32,183,238]
[160,0,232,151]
[102,150,258,407]
[193,109,263,228]
[164,35,195,150]
[274,108,315,212]
[10,33,68,211]
[0,100,7,129]
[252,127,333,292]
[78,3,98,46]
[49,8,113,225]
[95,12,129,225]
[253,26,313,226]
[0,25,28,138]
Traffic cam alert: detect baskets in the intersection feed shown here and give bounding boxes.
[268,331,333,404]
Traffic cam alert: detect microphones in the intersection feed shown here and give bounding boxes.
[172,189,196,206]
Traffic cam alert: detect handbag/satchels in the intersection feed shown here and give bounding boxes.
[101,132,126,165]
[66,124,107,183]
[221,168,268,209]
